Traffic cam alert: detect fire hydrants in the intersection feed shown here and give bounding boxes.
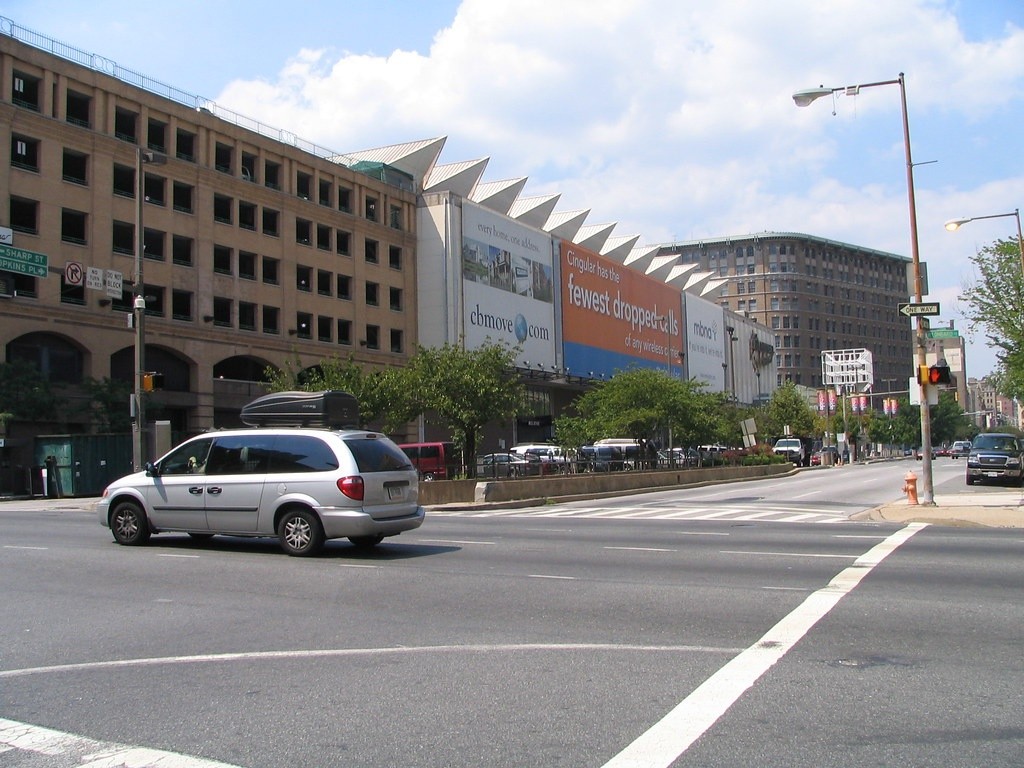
[902,470,919,505]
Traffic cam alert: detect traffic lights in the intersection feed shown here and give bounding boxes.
[929,365,951,385]
[143,373,165,392]
[916,364,930,387]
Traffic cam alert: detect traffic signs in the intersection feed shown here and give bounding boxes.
[919,316,930,332]
[0,243,49,279]
[896,302,940,317]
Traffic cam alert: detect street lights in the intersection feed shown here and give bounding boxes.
[653,309,674,464]
[790,72,936,507]
[130,145,171,471]
[943,208,1024,276]
[755,371,763,413]
[726,324,739,411]
[677,351,685,384]
[722,363,727,400]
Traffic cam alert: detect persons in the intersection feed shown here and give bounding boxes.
[189,455,206,474]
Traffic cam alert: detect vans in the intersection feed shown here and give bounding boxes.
[397,440,458,480]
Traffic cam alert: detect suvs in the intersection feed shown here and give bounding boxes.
[95,389,426,559]
[962,433,1024,487]
[772,438,805,468]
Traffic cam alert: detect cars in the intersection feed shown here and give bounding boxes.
[509,436,733,475]
[811,444,839,466]
[482,452,542,478]
[903,439,971,461]
